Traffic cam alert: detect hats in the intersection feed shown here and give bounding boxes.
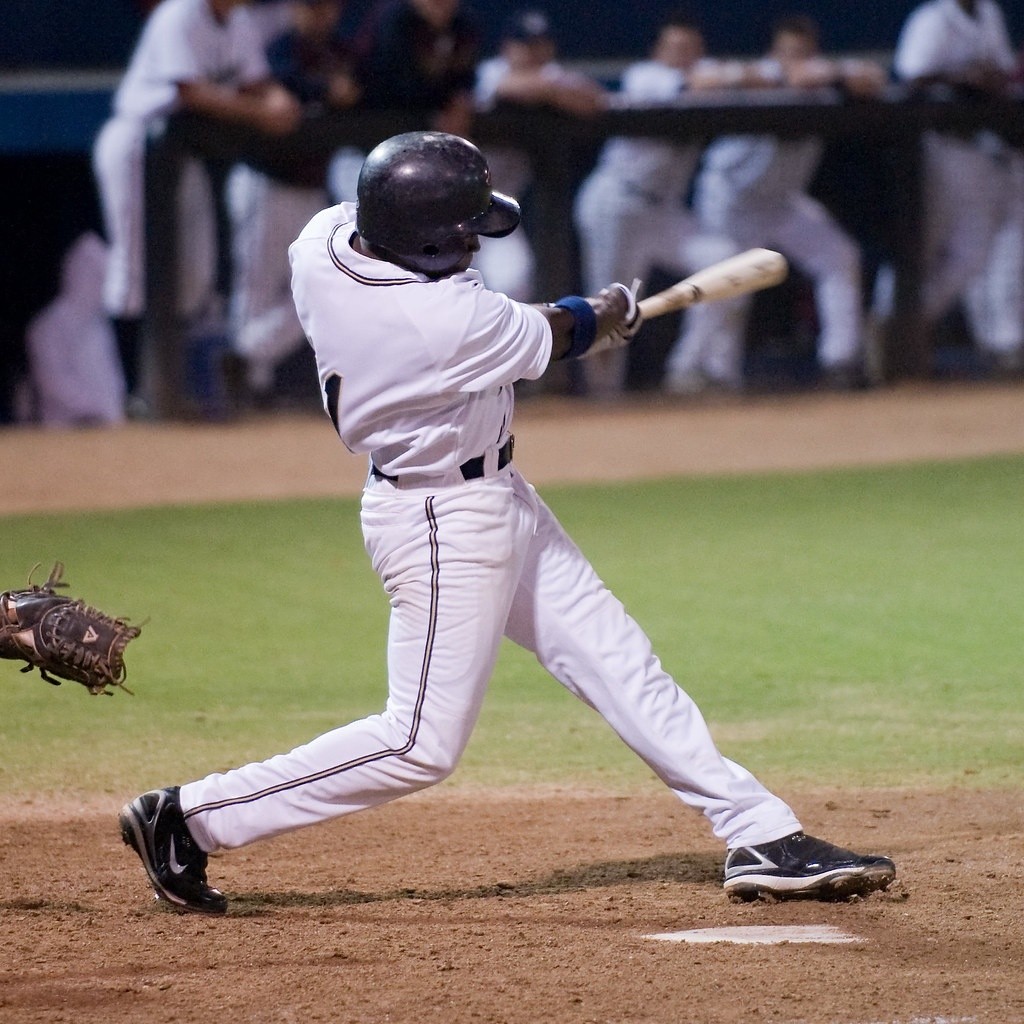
[508,6,562,46]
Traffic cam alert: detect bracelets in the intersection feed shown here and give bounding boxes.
[758,55,784,84]
[723,58,745,89]
[830,55,845,86]
[556,293,597,358]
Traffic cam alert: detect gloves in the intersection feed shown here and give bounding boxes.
[548,277,643,358]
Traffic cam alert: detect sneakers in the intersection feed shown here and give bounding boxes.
[723,830,898,905]
[116,785,229,914]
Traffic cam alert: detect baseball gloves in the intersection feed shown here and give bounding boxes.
[0,559,152,696]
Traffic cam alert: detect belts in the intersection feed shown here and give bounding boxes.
[368,431,517,480]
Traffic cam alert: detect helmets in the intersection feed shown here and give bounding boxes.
[354,130,522,274]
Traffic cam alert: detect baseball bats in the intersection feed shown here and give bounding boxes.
[603,247,787,342]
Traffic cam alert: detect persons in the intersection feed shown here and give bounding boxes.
[120,135,898,915]
[28,0,1024,435]
[1,560,138,694]
